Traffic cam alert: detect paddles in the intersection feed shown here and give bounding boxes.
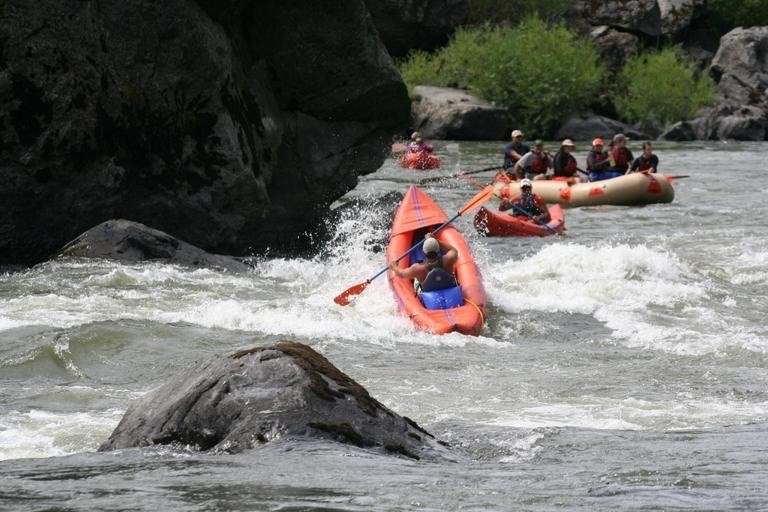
[334,184,493,306]
[463,174,563,235]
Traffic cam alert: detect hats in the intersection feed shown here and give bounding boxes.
[422,237,440,255]
[512,130,523,137]
[561,139,575,146]
[593,138,604,146]
[521,179,531,188]
[535,139,543,145]
[613,134,630,142]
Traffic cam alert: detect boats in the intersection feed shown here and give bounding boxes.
[490,171,678,206]
[399,150,443,171]
[384,183,489,338]
[473,202,566,238]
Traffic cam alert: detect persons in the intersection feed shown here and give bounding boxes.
[586,138,622,182]
[407,132,433,153]
[554,139,579,177]
[513,140,555,180]
[390,232,458,292]
[503,130,530,180]
[604,133,634,175]
[499,178,550,225]
[630,142,658,173]
